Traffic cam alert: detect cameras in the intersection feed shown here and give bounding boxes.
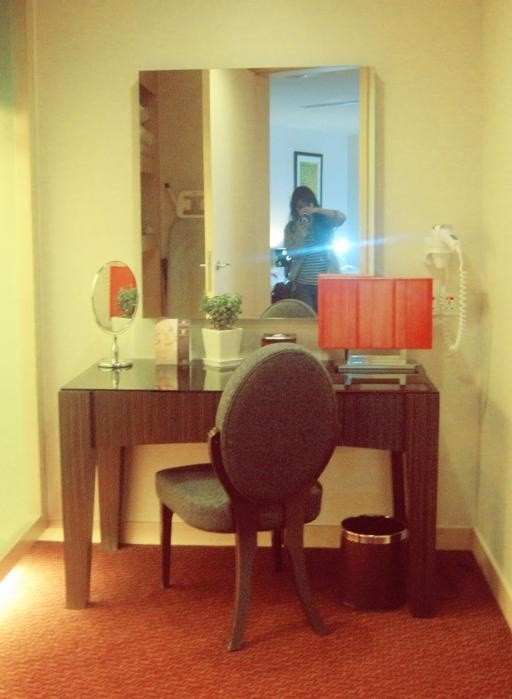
[301,214,312,224]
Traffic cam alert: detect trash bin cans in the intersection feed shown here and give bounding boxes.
[339,514,410,612]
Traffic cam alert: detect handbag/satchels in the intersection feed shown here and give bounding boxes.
[271,281,292,302]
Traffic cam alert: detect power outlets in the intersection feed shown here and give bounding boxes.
[431,291,460,316]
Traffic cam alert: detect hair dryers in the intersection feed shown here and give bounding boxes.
[424,225,462,270]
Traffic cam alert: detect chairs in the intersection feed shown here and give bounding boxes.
[259,298,317,320]
[158,347,337,653]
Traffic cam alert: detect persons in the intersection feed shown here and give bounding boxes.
[284,186,349,312]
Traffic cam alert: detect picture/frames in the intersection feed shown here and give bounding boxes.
[293,150,323,207]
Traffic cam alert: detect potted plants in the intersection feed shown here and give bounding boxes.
[200,290,243,363]
[110,287,138,332]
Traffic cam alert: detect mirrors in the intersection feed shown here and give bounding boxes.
[138,65,375,322]
[91,262,140,371]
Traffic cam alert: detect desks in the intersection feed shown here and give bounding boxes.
[57,354,441,620]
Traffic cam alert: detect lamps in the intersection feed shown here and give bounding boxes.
[317,274,435,386]
